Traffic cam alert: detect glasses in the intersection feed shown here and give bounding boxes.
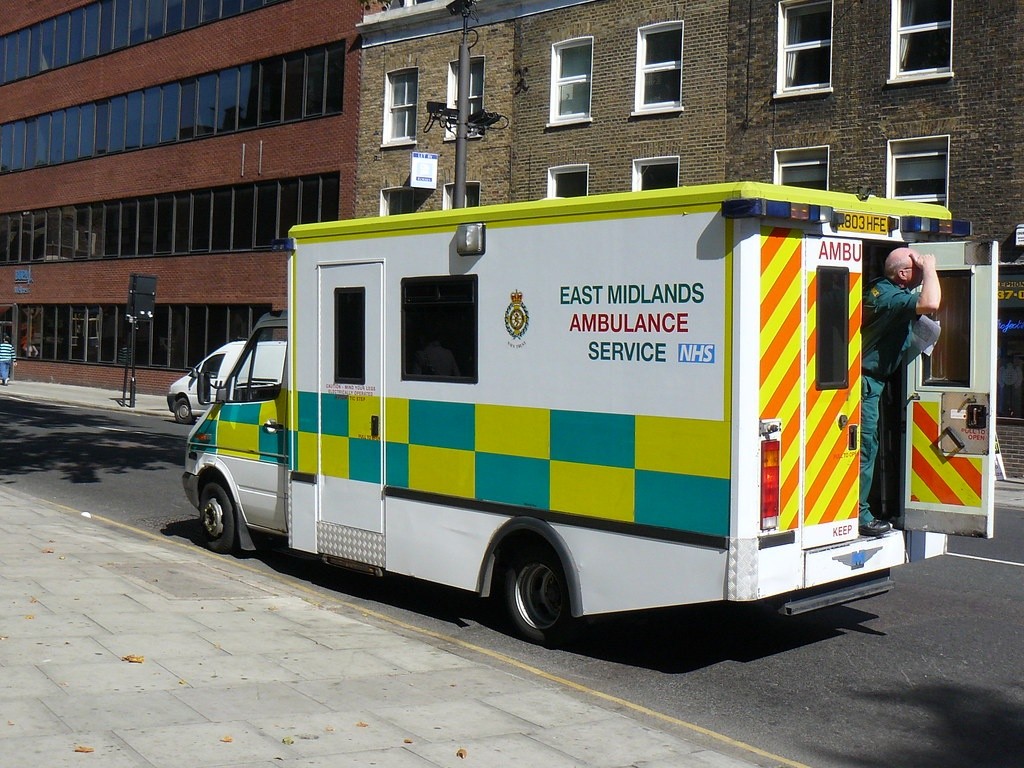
[903,266,920,272]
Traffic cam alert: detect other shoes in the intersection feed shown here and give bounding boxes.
[4,378,10,385]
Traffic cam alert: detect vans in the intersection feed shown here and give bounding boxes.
[166,339,288,423]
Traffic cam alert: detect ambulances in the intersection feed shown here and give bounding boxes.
[181,181,999,644]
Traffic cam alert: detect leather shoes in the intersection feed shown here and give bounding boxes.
[858,518,891,536]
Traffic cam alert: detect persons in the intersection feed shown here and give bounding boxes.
[24,345,39,357]
[858,247,941,536]
[0,336,18,386]
